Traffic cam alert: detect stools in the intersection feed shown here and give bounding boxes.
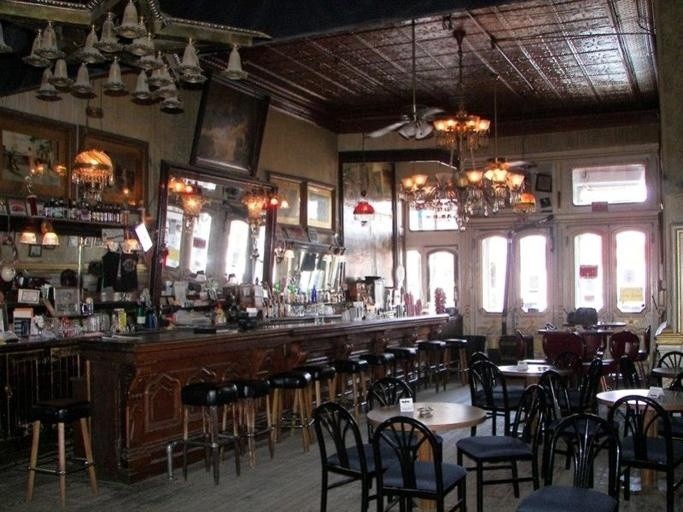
[24,398,98,507]
[219,379,275,471]
[290,365,336,436]
[266,370,315,452]
[180,382,240,485]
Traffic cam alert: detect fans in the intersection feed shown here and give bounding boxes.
[367,19,446,141]
[463,72,533,179]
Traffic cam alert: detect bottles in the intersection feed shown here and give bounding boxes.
[43,198,126,224]
[135,296,147,330]
[284,284,330,303]
[118,309,127,332]
[136,200,145,224]
[254,277,263,308]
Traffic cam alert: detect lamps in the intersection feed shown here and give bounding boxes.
[0,0,274,111]
[168,172,202,217]
[19,177,60,246]
[72,77,114,186]
[241,186,268,221]
[398,28,537,231]
[354,133,374,213]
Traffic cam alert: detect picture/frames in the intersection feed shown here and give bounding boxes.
[0,107,77,207]
[77,124,149,213]
[189,67,270,179]
[266,171,336,235]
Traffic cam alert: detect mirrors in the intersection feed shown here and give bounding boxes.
[151,159,278,306]
